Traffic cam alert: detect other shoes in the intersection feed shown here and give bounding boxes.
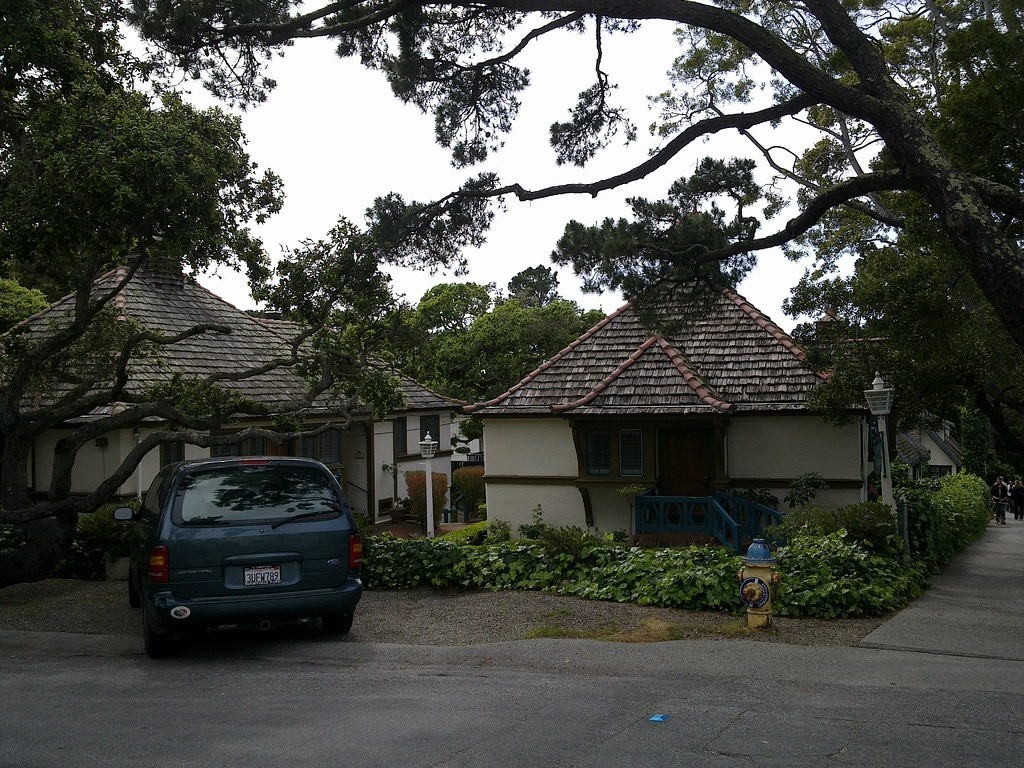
[1002,522,1007,524]
[998,522,1001,525]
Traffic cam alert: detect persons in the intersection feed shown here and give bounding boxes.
[990,476,1024,525]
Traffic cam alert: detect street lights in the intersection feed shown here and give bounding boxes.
[863,369,897,545]
[417,431,439,539]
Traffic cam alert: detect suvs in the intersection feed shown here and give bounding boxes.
[113,453,365,656]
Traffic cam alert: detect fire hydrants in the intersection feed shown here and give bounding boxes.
[737,535,781,627]
